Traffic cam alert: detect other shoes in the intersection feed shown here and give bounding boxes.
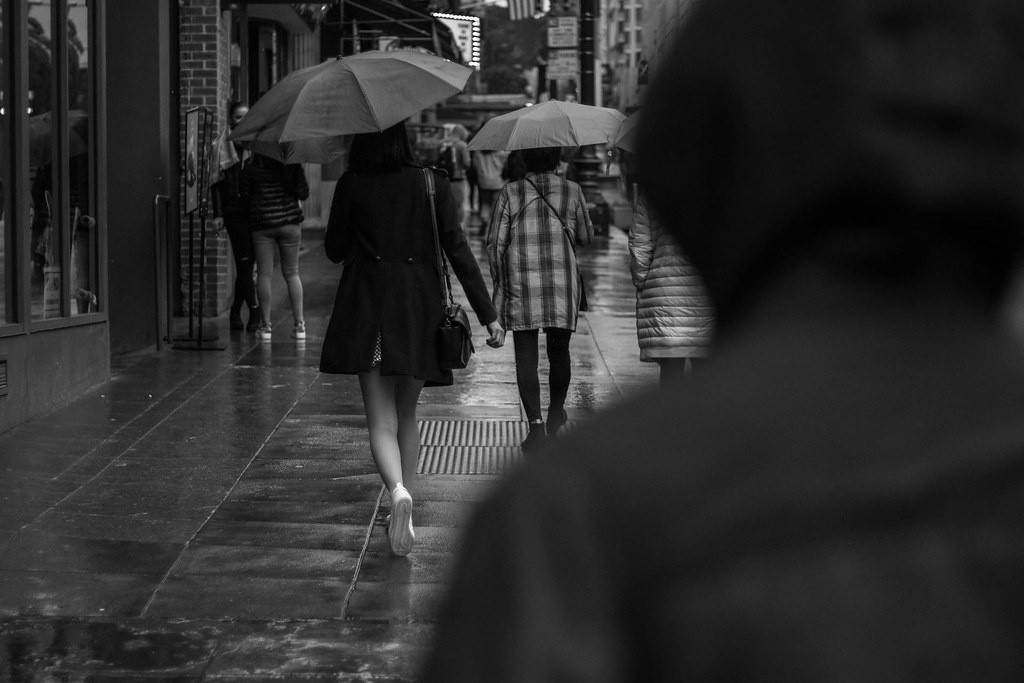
[478,221,488,236]
[385,484,415,555]
[291,323,306,339]
[256,323,273,341]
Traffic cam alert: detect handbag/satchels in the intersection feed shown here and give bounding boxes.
[437,302,475,368]
[579,273,589,311]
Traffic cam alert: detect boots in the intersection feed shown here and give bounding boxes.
[547,408,568,435]
[246,308,260,331]
[230,305,244,329]
[520,422,545,451]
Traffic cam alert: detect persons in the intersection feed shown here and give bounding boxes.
[486,146,594,443]
[214,103,309,339]
[629,192,715,391]
[438,123,503,228]
[320,122,503,554]
[425,0,1024,683]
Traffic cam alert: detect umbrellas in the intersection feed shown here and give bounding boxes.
[227,49,473,142]
[466,99,626,147]
[243,133,347,163]
